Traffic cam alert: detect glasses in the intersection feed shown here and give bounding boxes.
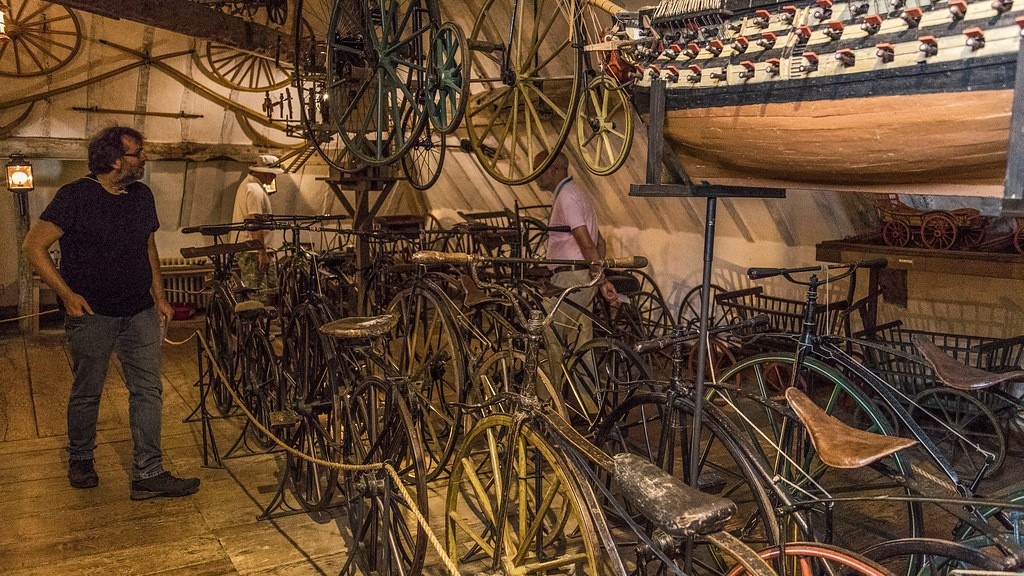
[122,147,147,159]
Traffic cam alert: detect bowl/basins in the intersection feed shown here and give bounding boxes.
[169,302,197,320]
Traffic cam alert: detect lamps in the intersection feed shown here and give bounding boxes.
[5,154,34,215]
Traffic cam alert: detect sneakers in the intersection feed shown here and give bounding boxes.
[130,470,201,500]
[67,457,99,488]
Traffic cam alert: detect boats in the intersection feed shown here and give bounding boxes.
[580,0,1023,219]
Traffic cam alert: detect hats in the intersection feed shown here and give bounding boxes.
[248,154,285,174]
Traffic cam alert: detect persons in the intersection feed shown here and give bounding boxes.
[231,156,285,309]
[23,125,201,502]
[532,149,620,433]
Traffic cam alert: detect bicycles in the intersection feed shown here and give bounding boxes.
[0,1,1024,576]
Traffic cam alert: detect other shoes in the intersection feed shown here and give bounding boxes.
[575,412,604,425]
[230,326,276,342]
[263,316,304,335]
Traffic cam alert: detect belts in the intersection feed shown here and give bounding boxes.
[550,264,589,277]
